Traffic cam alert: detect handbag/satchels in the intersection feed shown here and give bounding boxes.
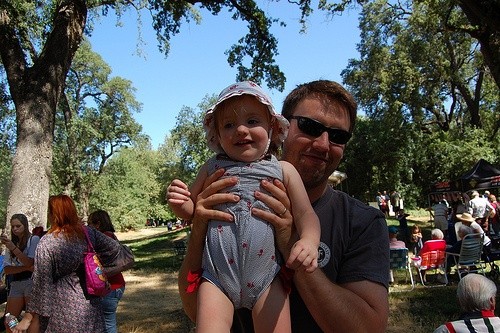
[81,225,112,297]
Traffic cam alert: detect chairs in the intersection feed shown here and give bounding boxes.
[389,231,486,292]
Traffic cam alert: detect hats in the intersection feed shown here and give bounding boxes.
[203,81,290,156]
[389,227,400,235]
[456,213,476,223]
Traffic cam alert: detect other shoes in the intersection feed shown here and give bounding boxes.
[389,282,395,287]
[405,280,417,284]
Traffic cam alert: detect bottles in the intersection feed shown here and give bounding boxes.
[5,313,19,333]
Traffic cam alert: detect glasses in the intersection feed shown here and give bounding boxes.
[288,116,352,145]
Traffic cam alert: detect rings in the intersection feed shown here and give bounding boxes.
[279,207,287,216]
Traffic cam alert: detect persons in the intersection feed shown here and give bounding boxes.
[178,80,391,333]
[168,217,181,231]
[0,194,125,333]
[376,191,500,286]
[435,274,500,333]
[166,81,321,333]
